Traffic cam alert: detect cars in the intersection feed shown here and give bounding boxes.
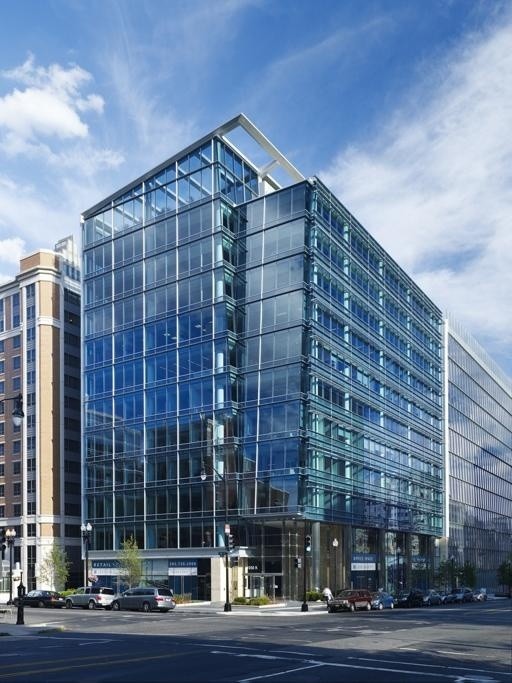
[15,586,176,613]
[327,587,488,613]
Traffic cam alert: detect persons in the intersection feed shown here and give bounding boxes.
[322,586,331,602]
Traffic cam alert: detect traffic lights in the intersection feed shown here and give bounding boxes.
[230,555,238,567]
[305,535,311,552]
[0,528,6,552]
[229,534,235,550]
[295,558,301,569]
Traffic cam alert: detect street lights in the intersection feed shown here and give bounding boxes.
[450,555,456,586]
[396,547,402,588]
[80,523,92,586]
[6,528,17,605]
[200,460,232,611]
[332,537,338,597]
